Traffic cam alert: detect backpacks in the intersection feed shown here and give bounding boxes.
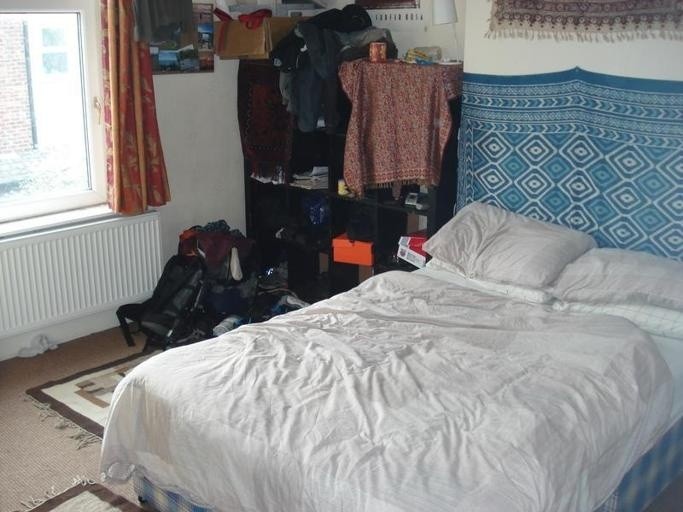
[115,253,210,353]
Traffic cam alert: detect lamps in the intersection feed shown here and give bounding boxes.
[431,0,462,66]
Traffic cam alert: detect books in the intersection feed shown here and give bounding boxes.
[287,164,330,191]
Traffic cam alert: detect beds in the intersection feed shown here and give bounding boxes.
[122,266,682,511]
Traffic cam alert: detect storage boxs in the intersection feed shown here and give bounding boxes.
[211,16,313,61]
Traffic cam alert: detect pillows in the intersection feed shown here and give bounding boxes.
[422,200,682,340]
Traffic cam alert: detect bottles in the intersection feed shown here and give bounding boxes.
[210,314,242,337]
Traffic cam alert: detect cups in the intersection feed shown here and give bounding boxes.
[369,42,387,63]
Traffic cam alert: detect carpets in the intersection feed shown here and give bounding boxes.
[15,475,145,511]
[23,345,172,440]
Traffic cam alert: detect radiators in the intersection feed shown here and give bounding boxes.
[0,211,162,339]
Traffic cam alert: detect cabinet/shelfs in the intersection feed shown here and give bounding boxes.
[231,57,463,309]
